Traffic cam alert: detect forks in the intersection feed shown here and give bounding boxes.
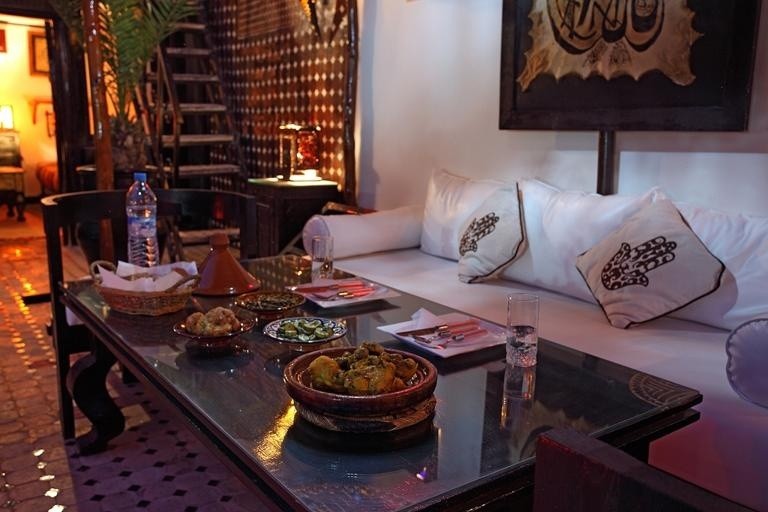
[427,329,488,350]
[310,291,371,301]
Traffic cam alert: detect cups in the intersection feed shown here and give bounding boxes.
[311,236,334,282]
[503,368,537,399]
[507,294,540,367]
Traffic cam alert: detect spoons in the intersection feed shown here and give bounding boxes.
[312,287,377,300]
[411,324,479,344]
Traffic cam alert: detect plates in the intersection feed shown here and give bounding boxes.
[283,346,438,416]
[173,315,256,341]
[175,341,254,371]
[284,277,402,309]
[263,317,348,344]
[376,312,513,358]
[235,291,306,314]
[281,422,435,475]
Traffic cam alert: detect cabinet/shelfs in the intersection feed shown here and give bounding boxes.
[247,175,338,259]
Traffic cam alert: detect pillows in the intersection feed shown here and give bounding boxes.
[574,200,726,328]
[457,181,526,283]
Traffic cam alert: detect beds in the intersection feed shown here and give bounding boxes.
[36,161,59,197]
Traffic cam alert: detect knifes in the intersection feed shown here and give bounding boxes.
[395,320,480,337]
[294,283,364,294]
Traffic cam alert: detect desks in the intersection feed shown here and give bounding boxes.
[0,166,26,222]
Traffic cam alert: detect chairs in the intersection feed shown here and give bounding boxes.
[39,186,270,440]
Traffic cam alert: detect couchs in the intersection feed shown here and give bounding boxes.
[300,167,768,507]
[527,422,758,512]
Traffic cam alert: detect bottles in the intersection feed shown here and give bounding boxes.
[126,172,159,267]
